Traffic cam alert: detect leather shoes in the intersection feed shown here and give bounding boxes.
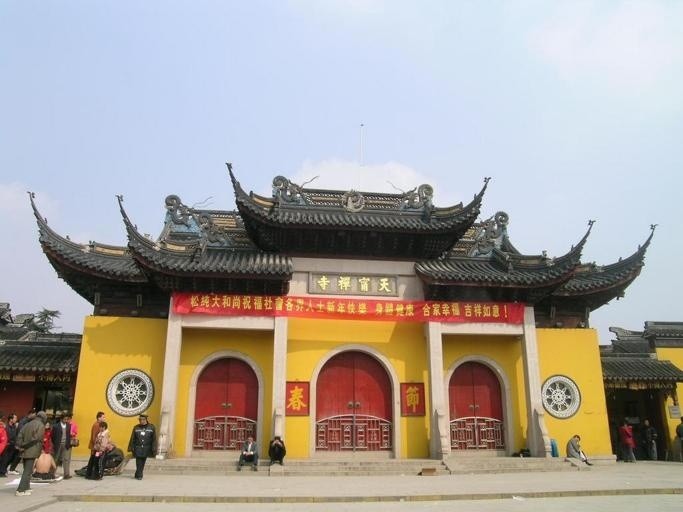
[0,474,7,477]
[587,463,593,465]
[64,474,72,479]
[138,476,141,480]
[238,462,283,471]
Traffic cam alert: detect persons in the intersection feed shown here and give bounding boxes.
[268,435,286,465]
[127,413,157,480]
[566,434,594,466]
[617,420,637,463]
[237,435,260,471]
[640,418,658,461]
[676,415,683,461]
[0,409,124,497]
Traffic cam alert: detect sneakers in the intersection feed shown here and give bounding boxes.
[26,489,32,495]
[9,470,19,474]
[16,488,25,496]
[75,469,103,480]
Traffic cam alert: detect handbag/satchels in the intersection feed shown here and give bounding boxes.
[70,436,80,447]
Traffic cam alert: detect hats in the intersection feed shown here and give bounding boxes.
[138,414,149,419]
